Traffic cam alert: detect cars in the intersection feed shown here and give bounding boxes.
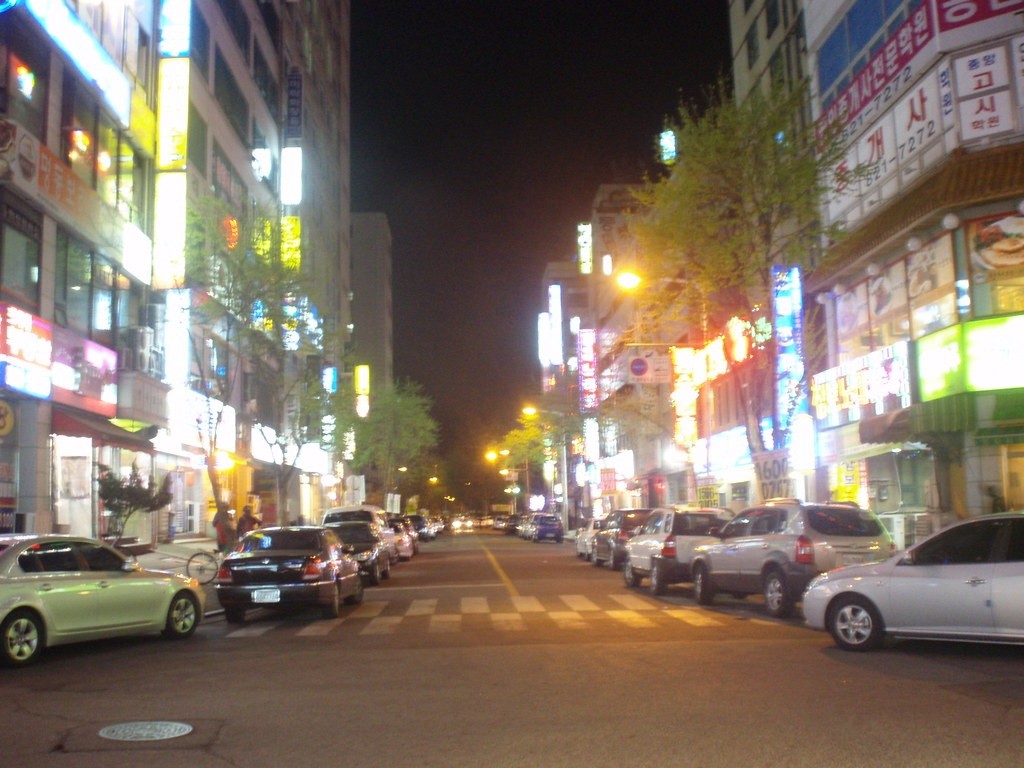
[575,519,604,561]
[390,515,444,559]
[802,512,1023,648]
[450,515,528,536]
[215,526,364,624]
[324,521,390,585]
[0,534,206,665]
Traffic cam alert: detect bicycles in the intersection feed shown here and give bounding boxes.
[186,548,223,585]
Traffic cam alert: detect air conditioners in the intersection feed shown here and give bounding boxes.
[186,501,206,534]
[878,514,912,551]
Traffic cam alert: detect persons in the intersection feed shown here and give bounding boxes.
[213,503,235,552]
[237,504,262,539]
[987,486,1006,513]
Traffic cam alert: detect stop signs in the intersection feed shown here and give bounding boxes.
[631,358,648,377]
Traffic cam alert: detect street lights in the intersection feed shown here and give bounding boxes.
[618,270,712,506]
[520,405,567,537]
[497,468,530,515]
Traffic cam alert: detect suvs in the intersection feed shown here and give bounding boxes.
[624,504,733,593]
[527,516,565,544]
[593,509,652,570]
[690,497,897,615]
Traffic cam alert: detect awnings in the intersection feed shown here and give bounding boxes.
[52,408,154,453]
[858,386,1024,462]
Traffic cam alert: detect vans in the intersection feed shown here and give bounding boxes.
[322,504,398,565]
